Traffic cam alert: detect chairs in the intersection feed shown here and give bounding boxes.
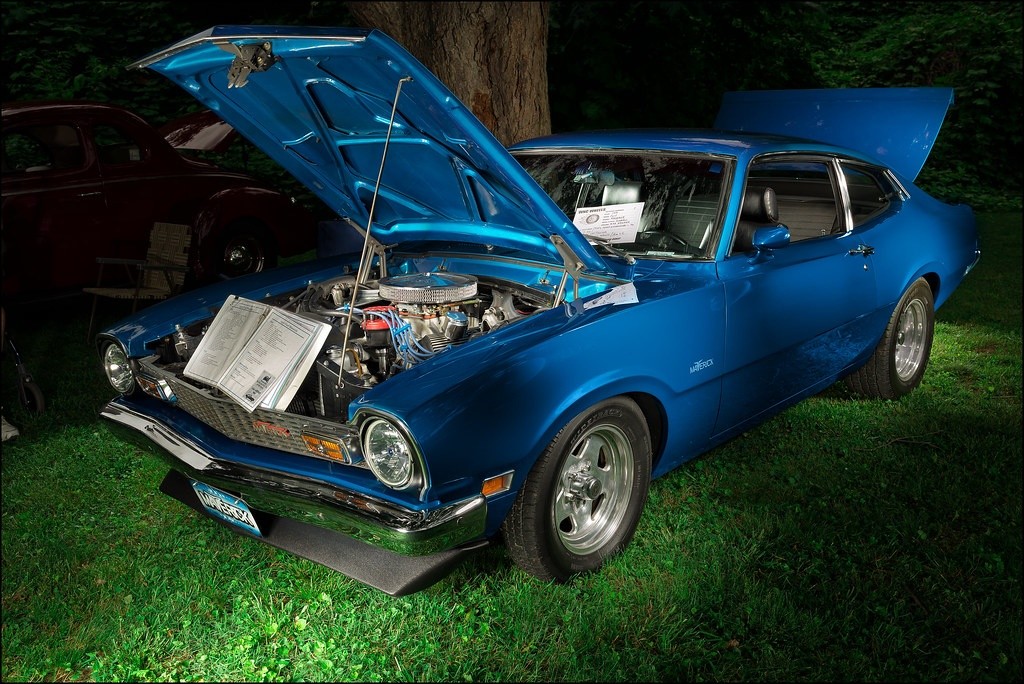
[82,221,192,345]
[733,186,778,252]
[35,125,82,167]
[602,179,645,206]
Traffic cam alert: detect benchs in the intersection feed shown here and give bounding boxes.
[672,196,837,250]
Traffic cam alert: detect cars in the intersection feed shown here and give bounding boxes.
[0,103,318,315]
[90,22,983,596]
